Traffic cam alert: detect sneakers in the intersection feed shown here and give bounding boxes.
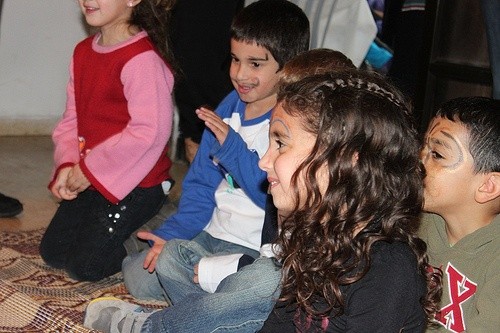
[84,296,162,333]
[0,194,23,217]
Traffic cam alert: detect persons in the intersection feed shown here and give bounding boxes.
[123,0,311,299]
[258,74,427,333]
[38,0,181,281]
[419,94,500,333]
[84,48,356,333]
